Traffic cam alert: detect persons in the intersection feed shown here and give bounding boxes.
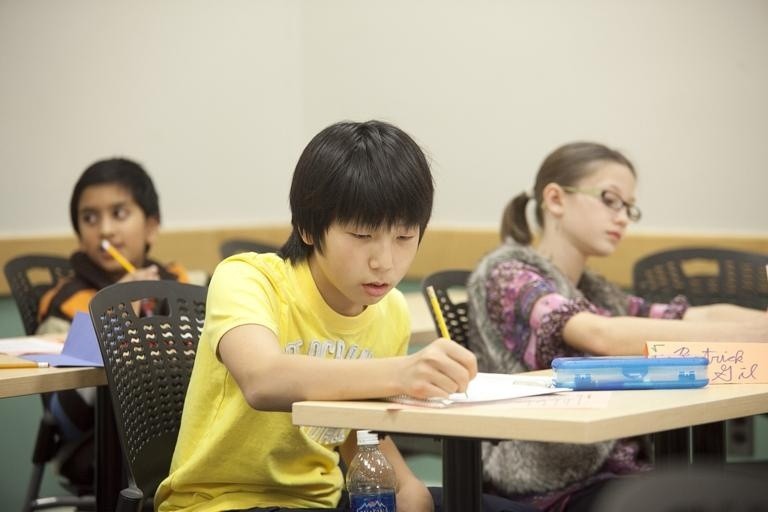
[462,142,768,509]
[149,119,479,510]
[33,156,216,510]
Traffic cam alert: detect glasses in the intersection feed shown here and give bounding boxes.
[540,184,642,223]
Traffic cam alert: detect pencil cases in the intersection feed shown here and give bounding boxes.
[551,357,709,390]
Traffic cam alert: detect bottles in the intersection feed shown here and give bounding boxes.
[345,430,398,512]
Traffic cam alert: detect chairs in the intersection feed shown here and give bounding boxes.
[5,251,87,511]
[630,246,766,311]
[421,268,507,512]
[87,280,208,512]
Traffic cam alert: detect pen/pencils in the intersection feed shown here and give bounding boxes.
[101,238,136,273]
[426,285,473,401]
[0,363,50,368]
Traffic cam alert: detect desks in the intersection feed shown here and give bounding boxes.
[1,287,468,512]
[292,369,767,512]
